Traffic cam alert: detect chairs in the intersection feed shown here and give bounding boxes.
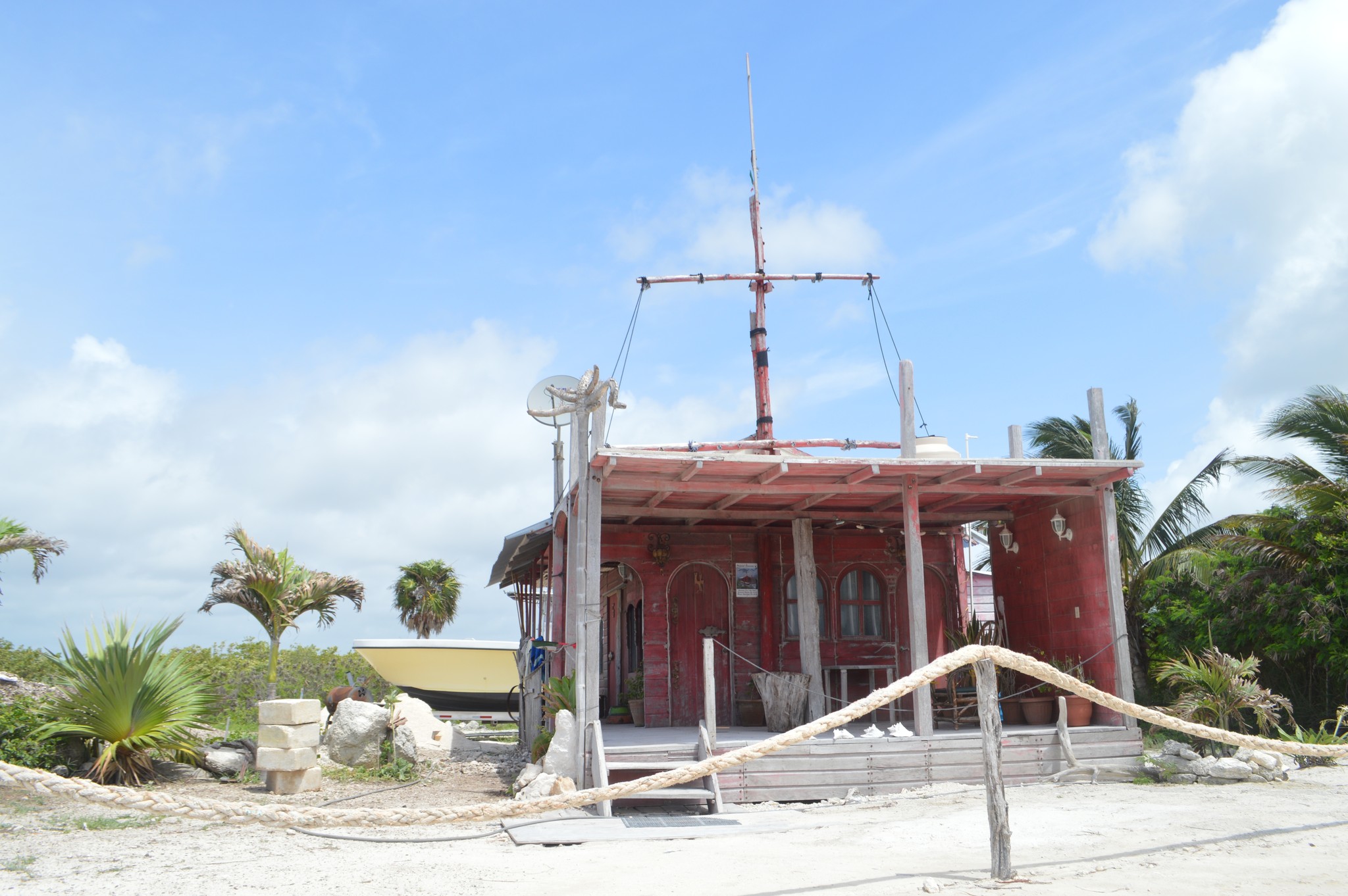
[930,665,1003,730]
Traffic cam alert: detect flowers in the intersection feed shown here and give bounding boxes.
[968,666,1018,699]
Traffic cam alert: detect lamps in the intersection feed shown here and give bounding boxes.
[1050,508,1073,541]
[998,523,1019,555]
[887,535,906,574]
[618,562,633,582]
[648,532,673,577]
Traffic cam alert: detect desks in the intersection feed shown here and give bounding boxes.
[821,664,899,726]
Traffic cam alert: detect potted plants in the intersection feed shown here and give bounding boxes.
[736,679,765,727]
[1024,642,1098,726]
[531,668,576,765]
[942,608,1000,686]
[617,659,646,727]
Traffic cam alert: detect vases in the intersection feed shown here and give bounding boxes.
[1000,696,1025,725]
[1017,696,1055,726]
